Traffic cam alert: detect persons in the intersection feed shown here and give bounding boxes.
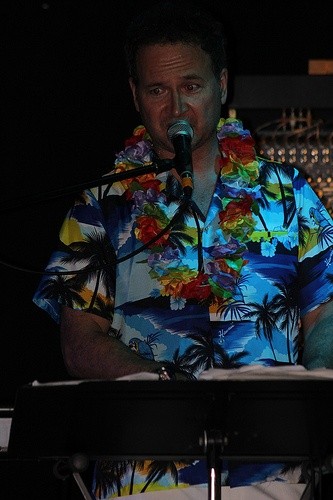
[32,7,332,500]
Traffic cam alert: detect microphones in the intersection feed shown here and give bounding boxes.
[165,119,194,203]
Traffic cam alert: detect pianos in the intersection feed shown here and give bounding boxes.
[5,365,333,465]
